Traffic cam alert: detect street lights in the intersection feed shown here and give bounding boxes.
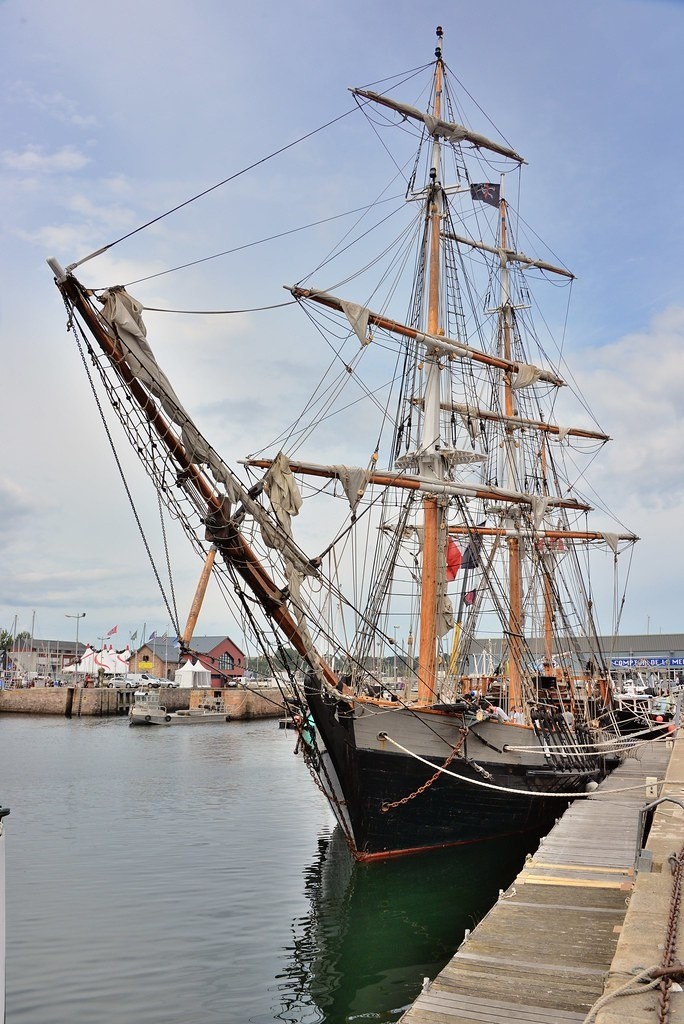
[96,636,111,687]
[64,612,86,686]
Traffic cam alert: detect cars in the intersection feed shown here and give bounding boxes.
[159,678,180,689]
[108,678,140,688]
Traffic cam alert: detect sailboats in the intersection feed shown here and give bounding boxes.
[40,21,676,856]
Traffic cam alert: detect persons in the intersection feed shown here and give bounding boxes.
[561,706,576,730]
[464,689,523,725]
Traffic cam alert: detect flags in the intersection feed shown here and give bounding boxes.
[130,631,138,641]
[161,631,168,644]
[106,624,118,636]
[149,630,156,643]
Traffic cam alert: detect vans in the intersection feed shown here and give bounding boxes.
[227,676,248,687]
[126,673,161,689]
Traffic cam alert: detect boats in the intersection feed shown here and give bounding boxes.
[130,691,165,714]
